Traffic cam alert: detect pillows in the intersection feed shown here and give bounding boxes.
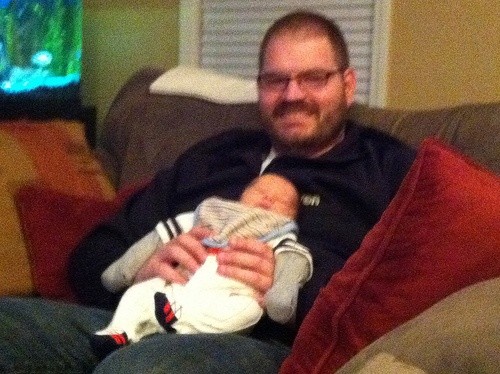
[1,119,118,300]
[278,137,500,374]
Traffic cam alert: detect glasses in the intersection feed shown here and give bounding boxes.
[255,68,335,94]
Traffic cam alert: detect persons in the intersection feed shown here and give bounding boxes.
[86,169,317,361]
[0,9,422,374]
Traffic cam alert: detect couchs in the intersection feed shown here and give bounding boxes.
[96,66,500,373]
